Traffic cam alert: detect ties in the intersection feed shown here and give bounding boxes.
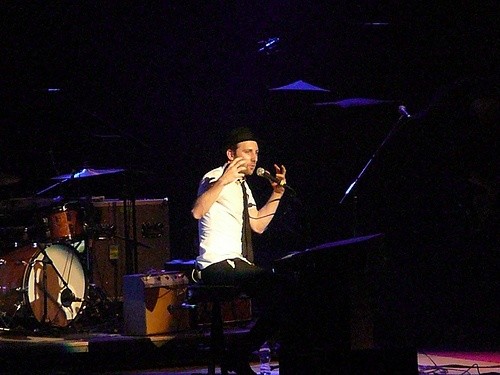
[238,179,254,262]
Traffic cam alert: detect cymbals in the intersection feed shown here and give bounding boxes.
[49,168,125,180]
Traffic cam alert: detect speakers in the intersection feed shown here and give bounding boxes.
[125,198,171,275]
[190,295,252,325]
[123,271,186,334]
[84,200,132,301]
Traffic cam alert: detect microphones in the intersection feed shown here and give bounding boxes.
[397,105,410,119]
[256,168,296,195]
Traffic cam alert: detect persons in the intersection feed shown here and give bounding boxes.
[191,128,286,375]
[15,198,47,244]
[437,97,500,212]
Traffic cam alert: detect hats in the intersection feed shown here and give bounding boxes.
[223,127,260,155]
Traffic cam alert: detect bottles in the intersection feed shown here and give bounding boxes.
[259,340,271,375]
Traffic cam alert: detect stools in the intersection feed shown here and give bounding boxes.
[186,285,245,375]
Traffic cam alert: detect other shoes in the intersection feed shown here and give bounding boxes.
[221,350,257,375]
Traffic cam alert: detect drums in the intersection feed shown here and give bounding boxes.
[0,243,91,332]
[43,205,90,243]
